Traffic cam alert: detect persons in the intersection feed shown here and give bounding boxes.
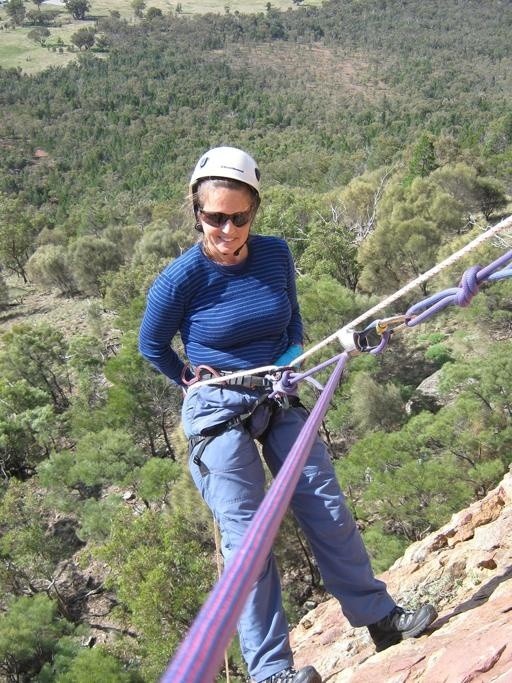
[138,146,438,683]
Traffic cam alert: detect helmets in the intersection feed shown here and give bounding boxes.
[189,146,261,208]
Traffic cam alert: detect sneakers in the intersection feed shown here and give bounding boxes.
[258,665,321,683]
[367,604,438,652]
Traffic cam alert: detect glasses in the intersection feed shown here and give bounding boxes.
[199,205,252,227]
